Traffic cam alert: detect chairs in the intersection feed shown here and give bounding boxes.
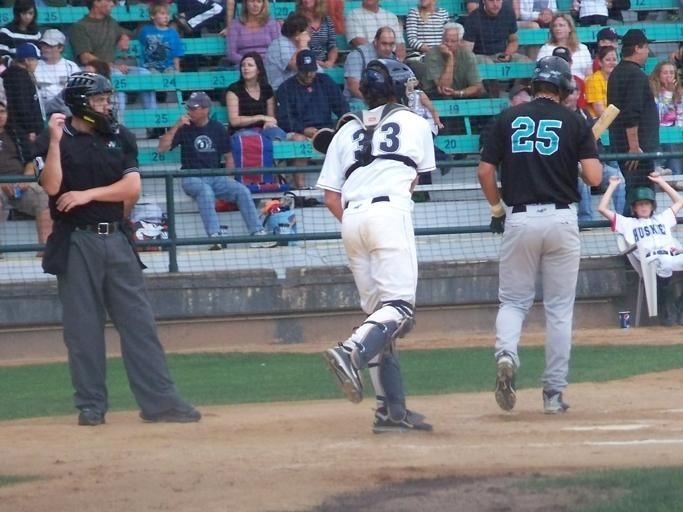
[617,235,683,327]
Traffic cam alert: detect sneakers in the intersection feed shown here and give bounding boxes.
[324,346,363,405]
[140,402,201,424]
[146,126,165,140]
[542,390,570,414]
[207,234,222,251]
[371,408,433,434]
[250,231,277,248]
[494,354,517,412]
[77,406,105,426]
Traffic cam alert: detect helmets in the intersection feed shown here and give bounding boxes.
[62,70,113,129]
[529,56,575,103]
[358,58,415,106]
[628,186,655,204]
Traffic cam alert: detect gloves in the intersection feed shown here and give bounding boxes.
[488,204,507,234]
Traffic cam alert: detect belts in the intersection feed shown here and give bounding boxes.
[75,221,122,236]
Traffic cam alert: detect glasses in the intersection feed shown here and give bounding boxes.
[569,86,578,94]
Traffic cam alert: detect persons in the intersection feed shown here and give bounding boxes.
[598,173,683,323]
[478,57,602,416]
[324,59,437,434]
[666,41,683,102]
[274,50,351,188]
[4,43,45,167]
[586,46,619,118]
[509,86,532,109]
[575,0,636,27]
[39,73,202,424]
[264,15,325,89]
[131,0,185,106]
[225,51,287,189]
[424,22,485,101]
[536,18,592,79]
[68,0,168,140]
[346,1,405,57]
[405,0,453,54]
[527,10,555,29]
[225,1,281,70]
[344,26,411,102]
[460,2,533,89]
[552,48,585,110]
[80,63,121,126]
[296,1,339,69]
[302,2,345,31]
[0,0,42,72]
[610,30,661,218]
[565,78,623,219]
[594,27,622,69]
[162,92,277,250]
[178,1,237,40]
[34,28,82,122]
[652,62,677,128]
[415,88,444,139]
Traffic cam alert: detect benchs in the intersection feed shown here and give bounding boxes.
[0,0,683,210]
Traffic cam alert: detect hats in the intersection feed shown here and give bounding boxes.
[508,85,528,99]
[180,92,211,109]
[621,28,656,44]
[596,28,621,42]
[296,49,318,73]
[552,46,571,62]
[16,41,48,62]
[39,28,65,47]
